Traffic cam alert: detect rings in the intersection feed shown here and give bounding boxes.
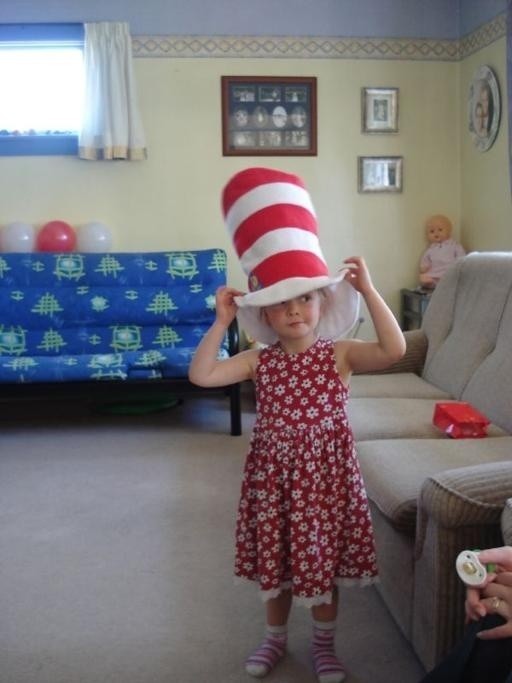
[491,596,502,614]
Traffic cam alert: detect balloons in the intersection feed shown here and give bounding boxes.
[74,222,113,254]
[37,220,76,252]
[0,220,34,254]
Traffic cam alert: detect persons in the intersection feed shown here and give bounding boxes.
[478,82,494,131]
[422,539,511,683]
[471,102,489,135]
[414,214,469,290]
[180,253,409,682]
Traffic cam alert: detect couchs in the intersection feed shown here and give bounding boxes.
[345,250,512,674]
[1,249,241,436]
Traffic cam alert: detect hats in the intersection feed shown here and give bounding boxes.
[218,167,362,344]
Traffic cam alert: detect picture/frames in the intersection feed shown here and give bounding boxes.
[466,65,501,153]
[222,76,317,156]
[362,86,399,133]
[359,156,404,193]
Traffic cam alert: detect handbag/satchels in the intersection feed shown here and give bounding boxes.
[434,403,491,438]
